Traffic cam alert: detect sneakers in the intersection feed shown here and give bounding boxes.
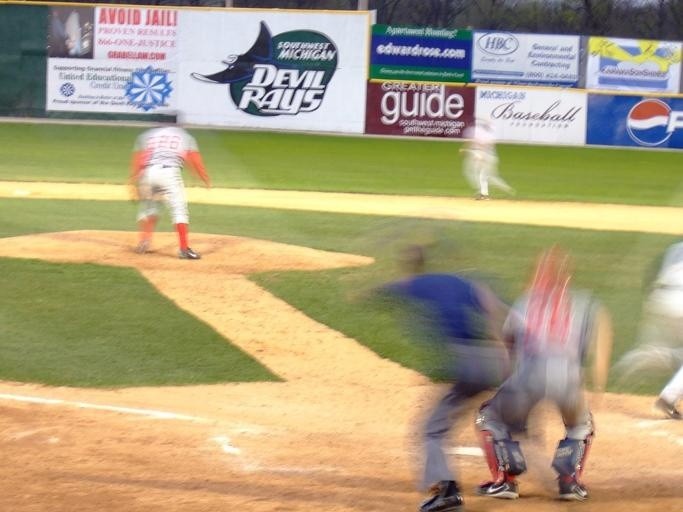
[476,195,490,201]
[654,396,683,419]
[555,473,591,502]
[474,478,520,500]
[418,479,465,512]
[178,247,201,259]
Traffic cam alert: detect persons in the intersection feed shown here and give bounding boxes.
[461,115,513,201]
[132,110,209,260]
[349,234,682,511]
